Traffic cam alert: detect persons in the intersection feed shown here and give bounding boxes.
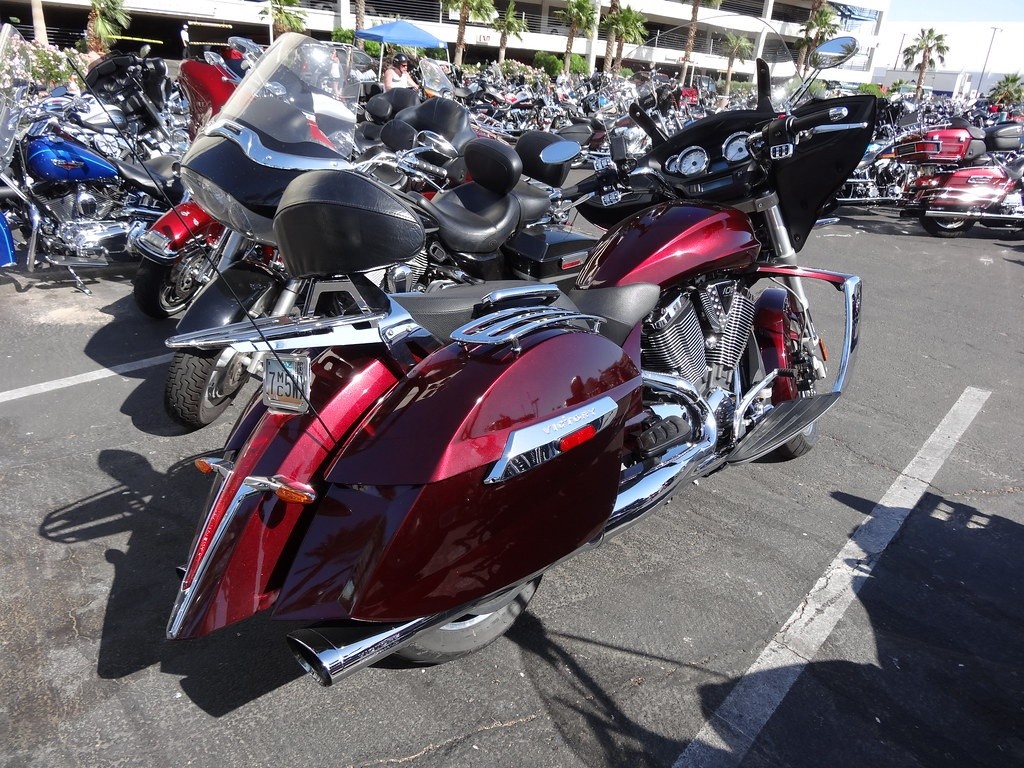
[384,54,420,92]
[556,68,570,86]
[180,24,192,61]
[994,97,1005,112]
[327,47,344,96]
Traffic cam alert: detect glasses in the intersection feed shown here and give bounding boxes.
[401,64,407,67]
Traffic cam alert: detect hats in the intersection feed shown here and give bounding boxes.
[183,25,188,29]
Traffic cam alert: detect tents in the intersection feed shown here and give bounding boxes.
[352,19,450,82]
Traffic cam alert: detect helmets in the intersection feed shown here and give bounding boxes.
[391,53,410,67]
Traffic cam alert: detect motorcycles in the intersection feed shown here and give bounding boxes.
[0,0,1024,416]
[167,8,883,692]
